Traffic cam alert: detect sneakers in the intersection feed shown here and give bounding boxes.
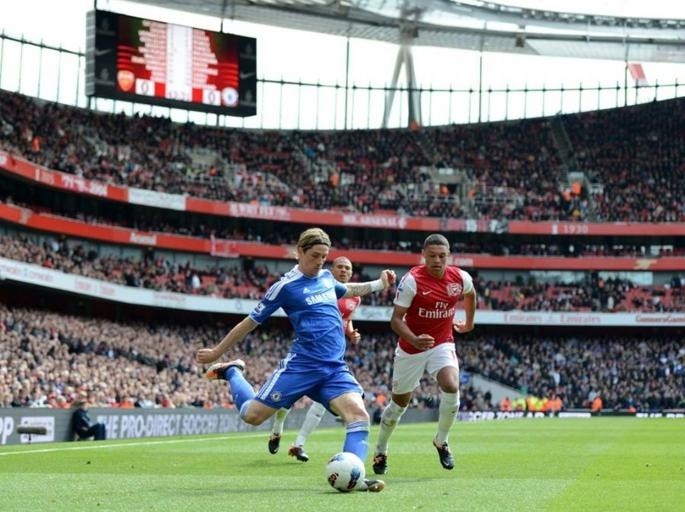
[207,359,245,379]
[374,448,388,474]
[433,433,454,470]
[269,433,281,454]
[357,479,384,492]
[290,446,308,461]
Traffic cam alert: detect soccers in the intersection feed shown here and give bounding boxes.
[327,452,364,491]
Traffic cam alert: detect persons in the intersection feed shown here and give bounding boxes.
[372,234,479,476]
[267,254,363,461]
[195,226,397,492]
[2,89,685,418]
[69,402,107,440]
